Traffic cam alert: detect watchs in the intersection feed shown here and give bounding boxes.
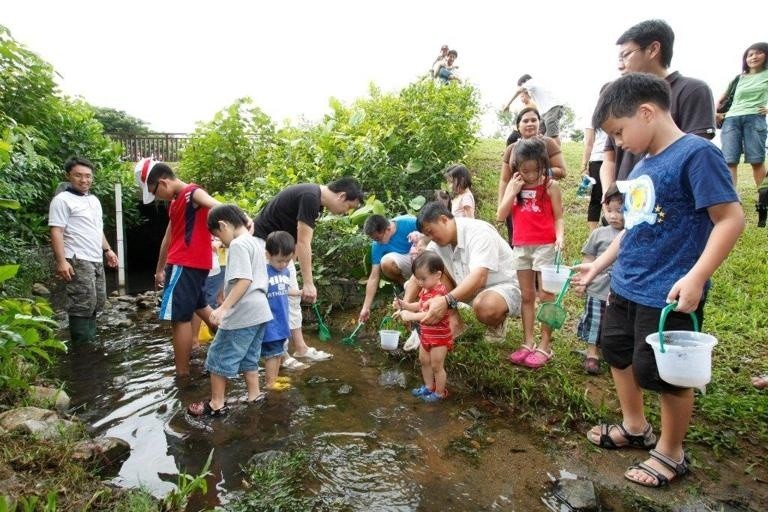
[445,292,457,309]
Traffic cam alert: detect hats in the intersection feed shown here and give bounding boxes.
[134,156,160,205]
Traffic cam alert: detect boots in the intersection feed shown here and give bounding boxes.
[67,315,103,354]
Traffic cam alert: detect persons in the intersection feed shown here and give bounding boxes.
[406,190,452,253]
[392,250,453,404]
[446,164,475,218]
[133,154,254,374]
[358,214,425,325]
[498,107,568,250]
[569,72,746,487]
[502,74,565,151]
[715,42,768,201]
[192,237,222,350]
[261,231,302,390]
[254,176,365,371]
[432,44,461,84]
[186,204,274,416]
[48,157,119,348]
[496,138,565,368]
[575,181,625,373]
[599,19,716,224]
[403,201,522,345]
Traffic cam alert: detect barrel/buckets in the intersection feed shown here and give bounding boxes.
[538,246,573,296]
[378,318,400,351]
[642,301,720,387]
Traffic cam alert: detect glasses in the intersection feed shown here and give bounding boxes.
[152,176,167,197]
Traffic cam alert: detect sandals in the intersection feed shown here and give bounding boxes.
[509,342,536,366]
[624,446,691,489]
[234,392,265,405]
[418,389,450,404]
[276,358,311,372]
[585,356,601,375]
[274,376,292,383]
[411,385,432,396]
[527,349,555,369]
[586,421,658,453]
[291,349,335,362]
[187,398,229,418]
[261,383,290,391]
